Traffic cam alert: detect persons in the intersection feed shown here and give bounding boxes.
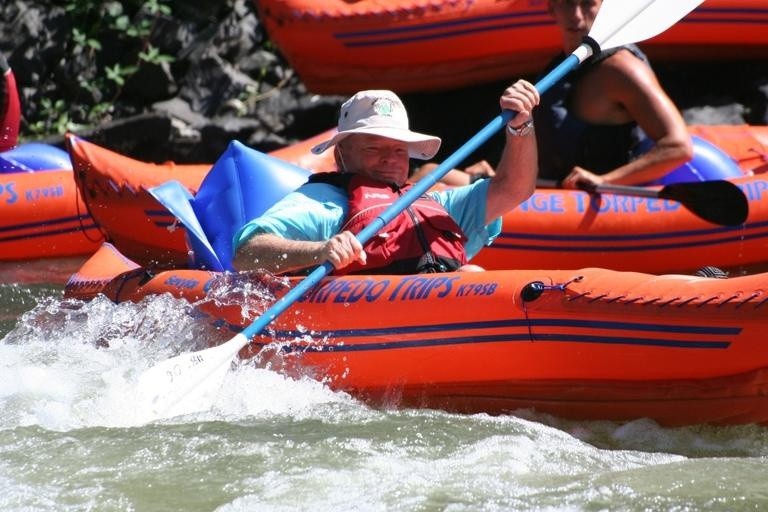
[233,79,540,275]
[534,0,694,190]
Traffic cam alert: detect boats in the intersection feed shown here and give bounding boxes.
[407,125,768,274]
[258,0,768,95]
[0,127,339,267]
[99,241,767,426]
[0,142,105,262]
[62,241,141,301]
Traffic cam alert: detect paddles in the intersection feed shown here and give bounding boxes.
[471,166,749,226]
[140,1,703,414]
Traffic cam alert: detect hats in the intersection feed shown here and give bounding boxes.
[312,89,441,161]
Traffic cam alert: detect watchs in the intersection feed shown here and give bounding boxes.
[507,120,533,138]
